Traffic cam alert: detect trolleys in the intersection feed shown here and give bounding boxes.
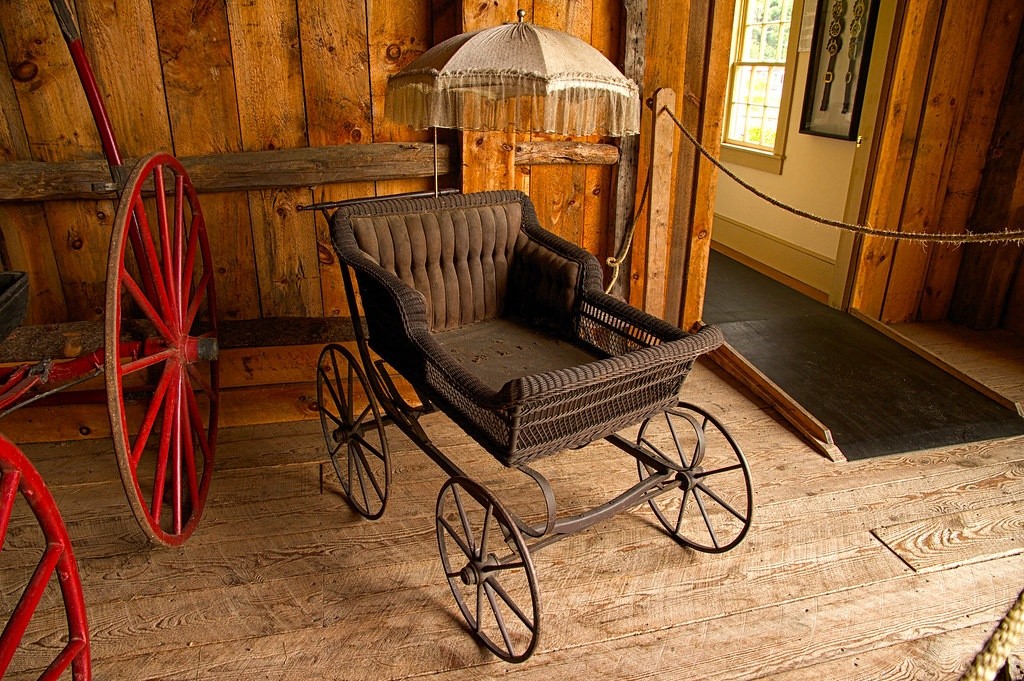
[297,9,753,663]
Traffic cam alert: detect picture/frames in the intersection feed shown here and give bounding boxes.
[797,0,883,142]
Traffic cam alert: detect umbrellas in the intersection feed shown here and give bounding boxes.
[383,10,641,199]
